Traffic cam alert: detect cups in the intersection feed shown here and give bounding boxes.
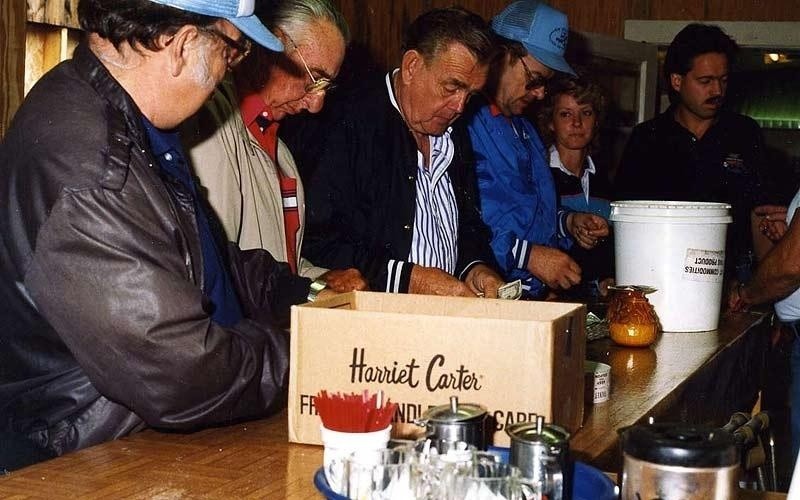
[322,422,391,496]
[345,396,573,500]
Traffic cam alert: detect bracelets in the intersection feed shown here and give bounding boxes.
[303,278,326,308]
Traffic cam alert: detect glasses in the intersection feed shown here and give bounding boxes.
[165,28,253,68]
[509,47,547,90]
[275,26,337,94]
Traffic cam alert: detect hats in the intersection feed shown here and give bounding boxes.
[148,1,284,52]
[491,0,579,78]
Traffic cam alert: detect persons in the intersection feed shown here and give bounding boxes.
[617,20,775,292]
[731,179,799,481]
[470,1,612,299]
[538,78,612,300]
[2,1,338,475]
[180,0,367,297]
[275,7,505,302]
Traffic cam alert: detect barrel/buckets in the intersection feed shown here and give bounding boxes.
[607,200,733,333]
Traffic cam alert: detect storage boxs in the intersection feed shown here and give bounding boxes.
[288,286,587,448]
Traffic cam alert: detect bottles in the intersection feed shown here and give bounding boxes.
[605,287,659,347]
